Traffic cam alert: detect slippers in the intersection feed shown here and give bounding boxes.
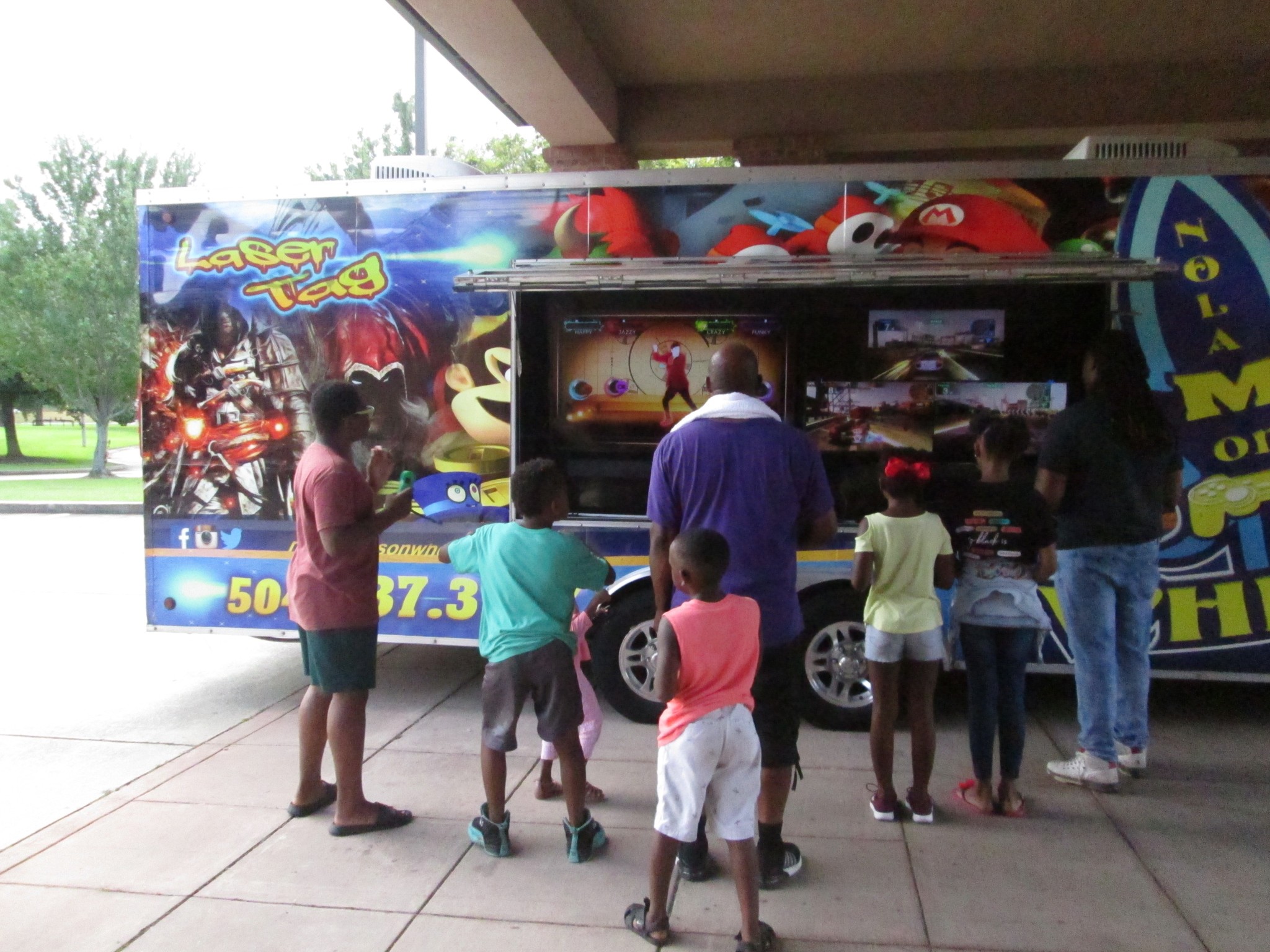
[952,780,994,816]
[287,780,337,817]
[330,801,414,836]
[1003,792,1026,818]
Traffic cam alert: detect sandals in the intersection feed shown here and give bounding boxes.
[735,920,776,952]
[625,897,670,945]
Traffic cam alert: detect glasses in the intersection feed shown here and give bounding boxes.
[343,405,375,421]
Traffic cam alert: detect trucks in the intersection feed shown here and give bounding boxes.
[139,149,1270,735]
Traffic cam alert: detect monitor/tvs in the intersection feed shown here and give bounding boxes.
[545,303,1078,467]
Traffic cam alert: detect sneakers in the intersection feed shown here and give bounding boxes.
[757,840,802,889]
[1114,744,1148,776]
[562,809,607,863]
[468,803,514,857]
[905,785,934,824]
[1047,748,1119,792]
[676,851,708,881]
[864,781,896,822]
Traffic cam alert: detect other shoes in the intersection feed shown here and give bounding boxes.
[583,781,606,803]
[534,780,563,798]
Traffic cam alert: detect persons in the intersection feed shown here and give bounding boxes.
[852,453,956,824]
[438,457,616,864]
[940,418,1060,817]
[1036,330,1183,792]
[625,529,776,952]
[285,379,414,837]
[534,588,611,801]
[649,342,837,888]
